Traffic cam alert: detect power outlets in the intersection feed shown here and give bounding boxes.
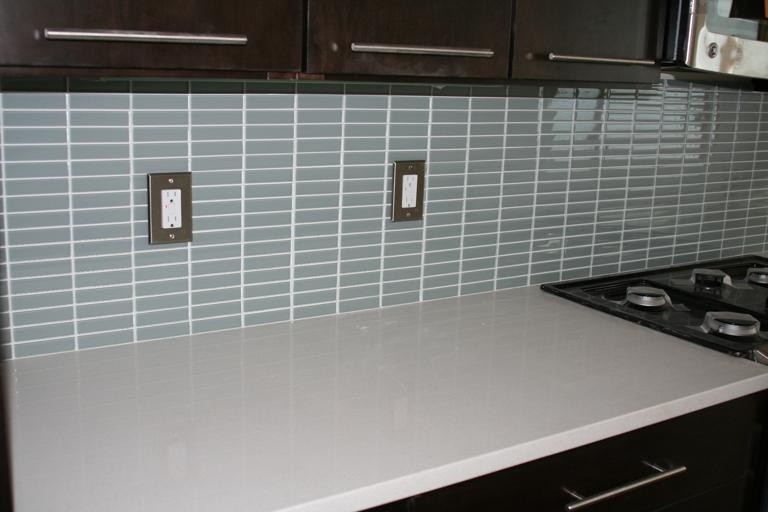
[144,171,192,246]
[390,159,425,222]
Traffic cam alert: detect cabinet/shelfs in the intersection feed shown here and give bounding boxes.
[361,391,766,512]
[304,1,514,95]
[510,1,670,98]
[0,0,305,85]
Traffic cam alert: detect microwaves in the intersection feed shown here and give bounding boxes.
[657,1,767,97]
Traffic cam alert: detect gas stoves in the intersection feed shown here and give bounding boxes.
[540,252,768,367]
